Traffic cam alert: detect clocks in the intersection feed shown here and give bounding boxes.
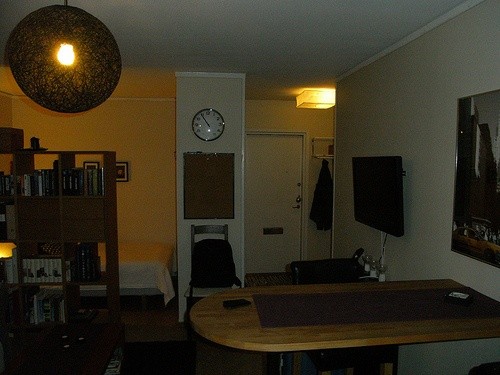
[192,109,225,142]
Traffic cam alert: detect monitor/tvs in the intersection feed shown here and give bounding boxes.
[352,157,405,238]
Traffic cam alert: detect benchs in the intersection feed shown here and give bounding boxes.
[80,240,176,314]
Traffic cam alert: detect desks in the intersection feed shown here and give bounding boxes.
[0,321,127,375]
[190,279,500,375]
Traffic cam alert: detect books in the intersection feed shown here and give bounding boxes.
[23,287,65,325]
[22,256,62,283]
[0,171,16,195]
[64,242,101,282]
[0,247,19,282]
[0,203,16,241]
[62,168,104,196]
[17,160,59,196]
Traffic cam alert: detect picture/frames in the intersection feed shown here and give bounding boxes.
[83,162,99,168]
[116,162,128,182]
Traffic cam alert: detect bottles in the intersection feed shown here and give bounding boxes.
[31,137,40,149]
[363,255,377,278]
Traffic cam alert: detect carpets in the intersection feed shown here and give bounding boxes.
[120,339,196,375]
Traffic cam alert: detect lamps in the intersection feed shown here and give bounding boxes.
[296,90,335,109]
[8,0,122,114]
[0,242,17,289]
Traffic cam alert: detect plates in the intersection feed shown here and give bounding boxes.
[17,148,48,152]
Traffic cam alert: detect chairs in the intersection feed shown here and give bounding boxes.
[185,224,232,308]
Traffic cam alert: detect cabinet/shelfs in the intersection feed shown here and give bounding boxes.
[0,151,120,320]
[290,258,398,374]
